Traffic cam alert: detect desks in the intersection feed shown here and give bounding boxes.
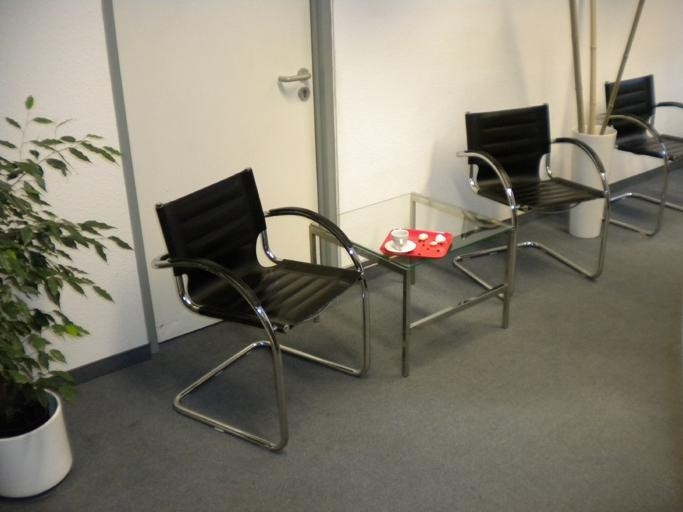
[308,190,515,379]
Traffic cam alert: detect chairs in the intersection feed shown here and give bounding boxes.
[604,73,683,237]
[452,106,611,303]
[152,165,373,453]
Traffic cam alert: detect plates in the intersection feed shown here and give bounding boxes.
[385,241,416,253]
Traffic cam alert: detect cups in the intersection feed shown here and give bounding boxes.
[392,230,408,250]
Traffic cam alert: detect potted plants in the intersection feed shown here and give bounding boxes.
[1,93,135,499]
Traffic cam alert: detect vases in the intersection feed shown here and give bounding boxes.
[567,124,618,239]
